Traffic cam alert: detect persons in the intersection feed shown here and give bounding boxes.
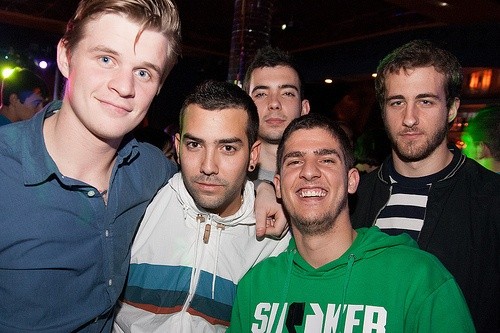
[242,47,310,180]
[110,81,294,333]
[461,107,500,175]
[0,69,50,127]
[122,127,177,169]
[226,112,477,333]
[347,39,499,333]
[0,0,290,333]
